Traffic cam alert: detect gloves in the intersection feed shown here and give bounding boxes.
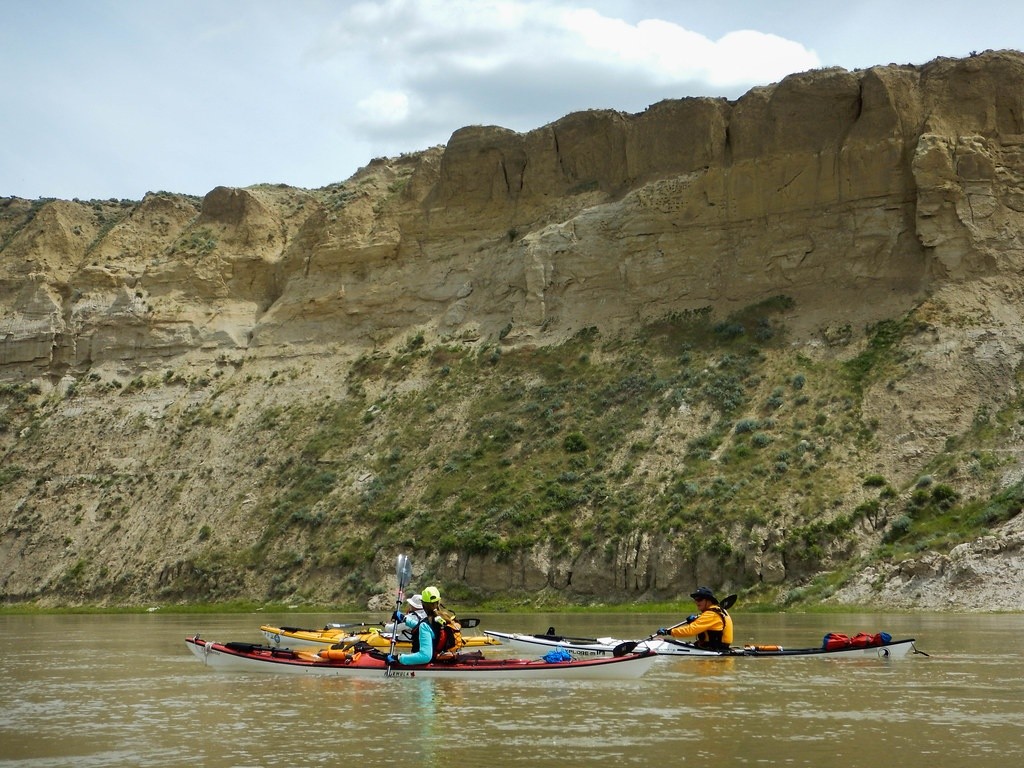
[686,614,697,623]
[657,627,670,635]
[385,654,398,666]
[391,611,406,624]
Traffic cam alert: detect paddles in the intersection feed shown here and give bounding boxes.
[386,553,412,678]
[326,618,481,629]
[612,594,737,658]
[225,641,293,655]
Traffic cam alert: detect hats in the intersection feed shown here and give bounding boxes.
[406,595,423,609]
[690,587,718,604]
[419,587,441,603]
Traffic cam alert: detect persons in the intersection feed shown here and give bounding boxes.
[382,594,427,642]
[656,586,733,651]
[384,586,461,666]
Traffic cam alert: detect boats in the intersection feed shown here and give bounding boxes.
[185,634,673,682]
[259,625,501,655]
[484,625,931,661]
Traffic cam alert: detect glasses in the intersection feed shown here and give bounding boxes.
[695,596,706,602]
